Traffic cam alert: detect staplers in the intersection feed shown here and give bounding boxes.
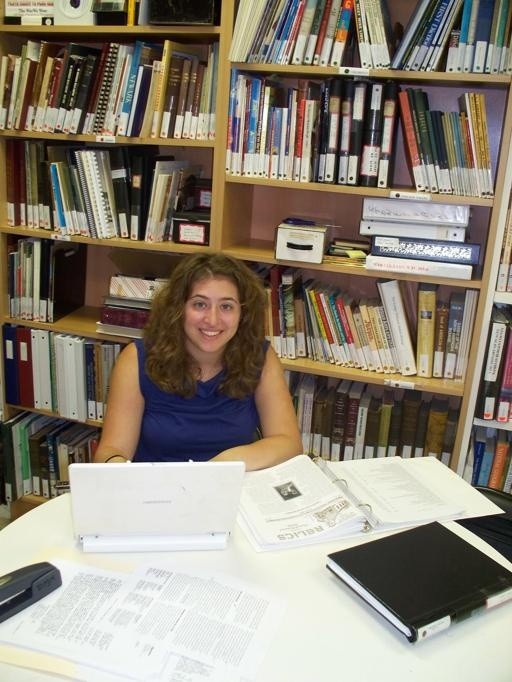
[2,562,61,623]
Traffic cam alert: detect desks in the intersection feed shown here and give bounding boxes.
[0,492,512,682]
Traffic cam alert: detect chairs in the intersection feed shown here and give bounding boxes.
[88,425,262,465]
[455,484,512,562]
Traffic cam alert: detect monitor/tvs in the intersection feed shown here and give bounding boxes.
[68,462,245,552]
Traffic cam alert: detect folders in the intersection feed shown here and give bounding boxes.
[226,70,452,198]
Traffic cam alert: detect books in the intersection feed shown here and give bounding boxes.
[225,67,494,198]
[283,370,461,469]
[97,274,169,339]
[4,0,150,26]
[462,425,512,494]
[475,304,512,422]
[228,0,512,75]
[234,456,466,551]
[0,136,203,244]
[6,237,88,323]
[245,261,480,384]
[0,39,216,140]
[0,409,103,502]
[495,209,512,292]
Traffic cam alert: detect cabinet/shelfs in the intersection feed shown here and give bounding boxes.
[0,0,511,530]
